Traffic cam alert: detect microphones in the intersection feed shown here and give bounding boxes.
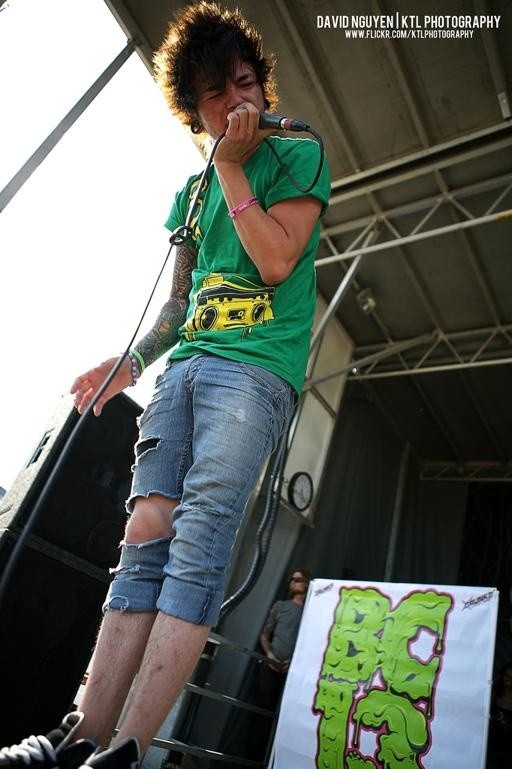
[234,104,310,132]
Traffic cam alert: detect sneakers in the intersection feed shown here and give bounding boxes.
[77,734,142,769]
[0,710,85,768]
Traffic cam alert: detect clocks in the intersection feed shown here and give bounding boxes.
[287,471,314,512]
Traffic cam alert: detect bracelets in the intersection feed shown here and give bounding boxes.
[130,349,147,371]
[227,196,260,221]
[124,352,138,387]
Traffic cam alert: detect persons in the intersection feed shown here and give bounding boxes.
[1,0,337,769]
[239,565,314,762]
